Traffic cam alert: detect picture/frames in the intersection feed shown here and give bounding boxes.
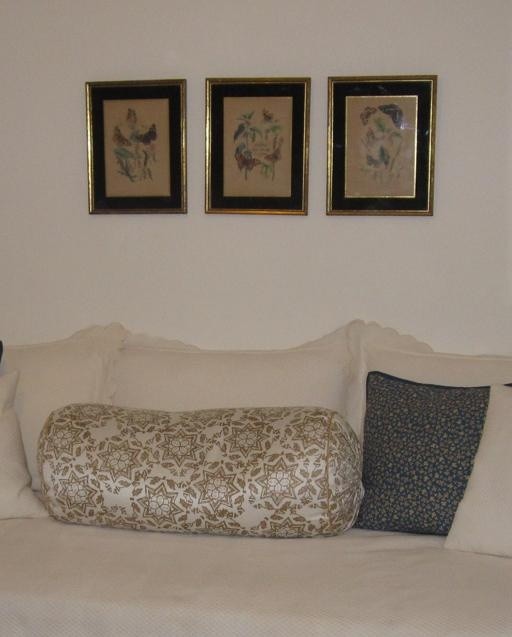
[84,79,188,215]
[206,77,311,216]
[326,75,434,217]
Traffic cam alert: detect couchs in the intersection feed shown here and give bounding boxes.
[0,517,511,636]
